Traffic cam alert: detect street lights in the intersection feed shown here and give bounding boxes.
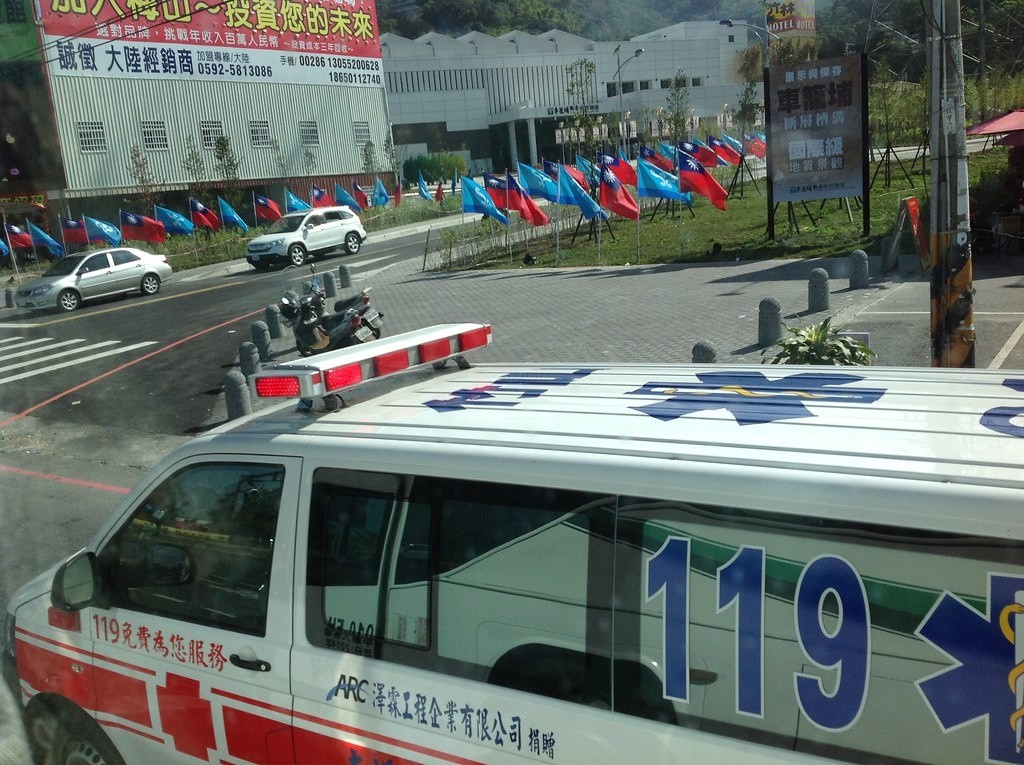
[609,44,647,163]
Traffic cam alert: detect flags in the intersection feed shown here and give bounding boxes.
[313,184,338,208]
[156,206,194,236]
[5,223,45,248]
[336,184,362,211]
[61,219,102,242]
[0,238,9,256]
[435,181,443,201]
[254,193,284,224]
[354,182,373,208]
[418,174,434,202]
[85,217,121,247]
[373,179,389,206]
[286,190,311,212]
[484,129,767,226]
[394,182,401,208]
[28,221,65,256]
[121,211,164,242]
[462,176,507,225]
[220,197,248,232]
[190,199,221,232]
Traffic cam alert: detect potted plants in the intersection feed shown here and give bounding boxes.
[760,315,879,367]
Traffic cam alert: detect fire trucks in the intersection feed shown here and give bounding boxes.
[1,320,1024,765]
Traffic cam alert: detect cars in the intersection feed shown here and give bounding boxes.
[11,246,173,314]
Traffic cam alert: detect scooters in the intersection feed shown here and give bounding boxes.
[280,264,385,356]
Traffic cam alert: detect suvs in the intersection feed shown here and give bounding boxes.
[245,204,367,271]
